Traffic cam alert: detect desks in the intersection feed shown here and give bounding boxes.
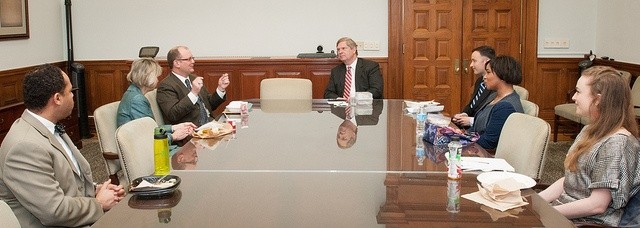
[84,99,579,228]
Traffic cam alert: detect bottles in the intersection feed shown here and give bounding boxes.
[416,104,426,134]
[449,135,463,181]
[416,135,426,167]
[153,128,170,176]
[446,180,462,219]
[157,209,172,224]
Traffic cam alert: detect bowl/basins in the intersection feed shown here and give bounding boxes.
[129,175,181,199]
[128,190,181,209]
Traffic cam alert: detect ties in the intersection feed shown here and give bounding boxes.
[185,79,208,126]
[343,65,352,99]
[470,82,486,110]
[344,105,352,120]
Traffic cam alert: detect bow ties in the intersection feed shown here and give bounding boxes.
[55,122,66,135]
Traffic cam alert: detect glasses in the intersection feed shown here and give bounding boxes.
[177,56,195,61]
[54,87,81,97]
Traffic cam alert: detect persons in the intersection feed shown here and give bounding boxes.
[0,63,125,228]
[466,55,525,149]
[156,47,230,128]
[537,66,640,228]
[450,45,495,129]
[324,37,384,100]
[331,101,383,149]
[171,143,198,170]
[117,58,197,157]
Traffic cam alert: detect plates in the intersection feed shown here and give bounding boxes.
[476,171,536,190]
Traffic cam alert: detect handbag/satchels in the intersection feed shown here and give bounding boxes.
[423,121,480,146]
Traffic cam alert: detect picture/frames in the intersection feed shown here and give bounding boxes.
[0,0,30,41]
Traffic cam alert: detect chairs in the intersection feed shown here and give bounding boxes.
[631,76,640,119]
[260,78,312,99]
[143,88,166,127]
[94,100,128,198]
[553,69,632,142]
[115,117,159,186]
[260,99,312,113]
[0,200,21,228]
[521,101,539,116]
[495,112,552,178]
[512,85,529,100]
